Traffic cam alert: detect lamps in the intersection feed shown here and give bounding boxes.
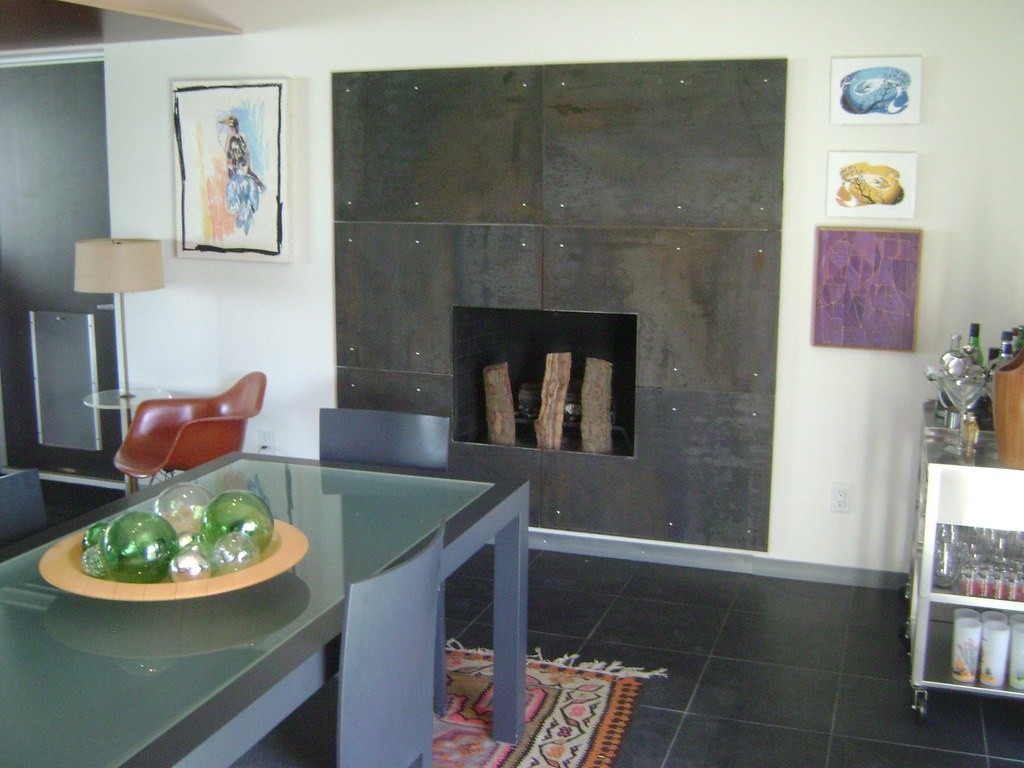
[73,235,169,395]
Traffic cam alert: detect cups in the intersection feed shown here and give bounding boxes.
[933,521,1024,694]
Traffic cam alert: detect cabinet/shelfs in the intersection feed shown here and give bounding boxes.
[906,403,1024,710]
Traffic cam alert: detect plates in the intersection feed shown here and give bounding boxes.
[37,508,310,604]
[45,572,315,658]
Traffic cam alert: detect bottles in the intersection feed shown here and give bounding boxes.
[937,321,1023,422]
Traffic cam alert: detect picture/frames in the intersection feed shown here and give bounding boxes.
[809,226,924,353]
[172,79,284,260]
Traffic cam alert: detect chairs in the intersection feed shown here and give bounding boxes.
[1,466,43,546]
[114,370,266,496]
[318,406,450,469]
[234,510,445,768]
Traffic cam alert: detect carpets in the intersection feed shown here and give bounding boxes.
[434,637,670,768]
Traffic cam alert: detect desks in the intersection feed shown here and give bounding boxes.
[0,452,543,768]
[83,388,170,493]
